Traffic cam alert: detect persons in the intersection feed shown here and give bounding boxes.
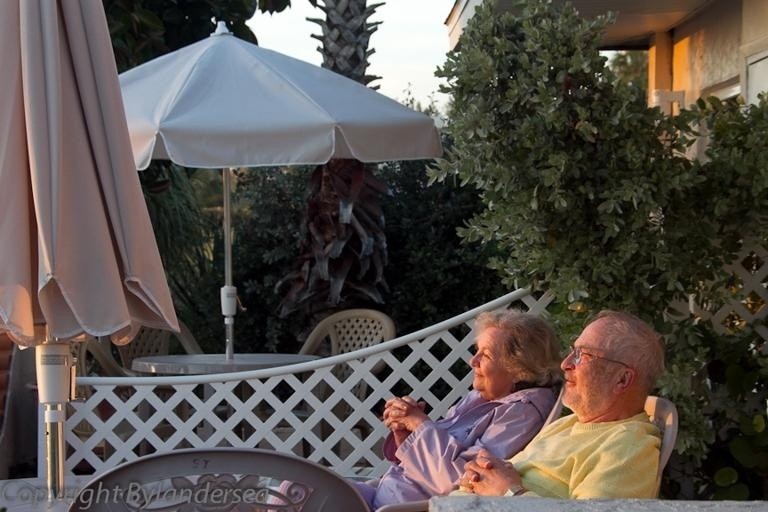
[374,309,665,512]
[267,311,563,512]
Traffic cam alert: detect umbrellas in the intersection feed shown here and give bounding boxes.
[0,0,182,502]
[117,18,445,359]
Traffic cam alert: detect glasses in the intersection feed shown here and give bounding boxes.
[569,345,629,369]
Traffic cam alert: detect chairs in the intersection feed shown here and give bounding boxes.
[541,379,678,488]
[299,309,395,451]
[63,446,368,510]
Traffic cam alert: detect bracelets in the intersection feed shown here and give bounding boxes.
[501,485,525,498]
